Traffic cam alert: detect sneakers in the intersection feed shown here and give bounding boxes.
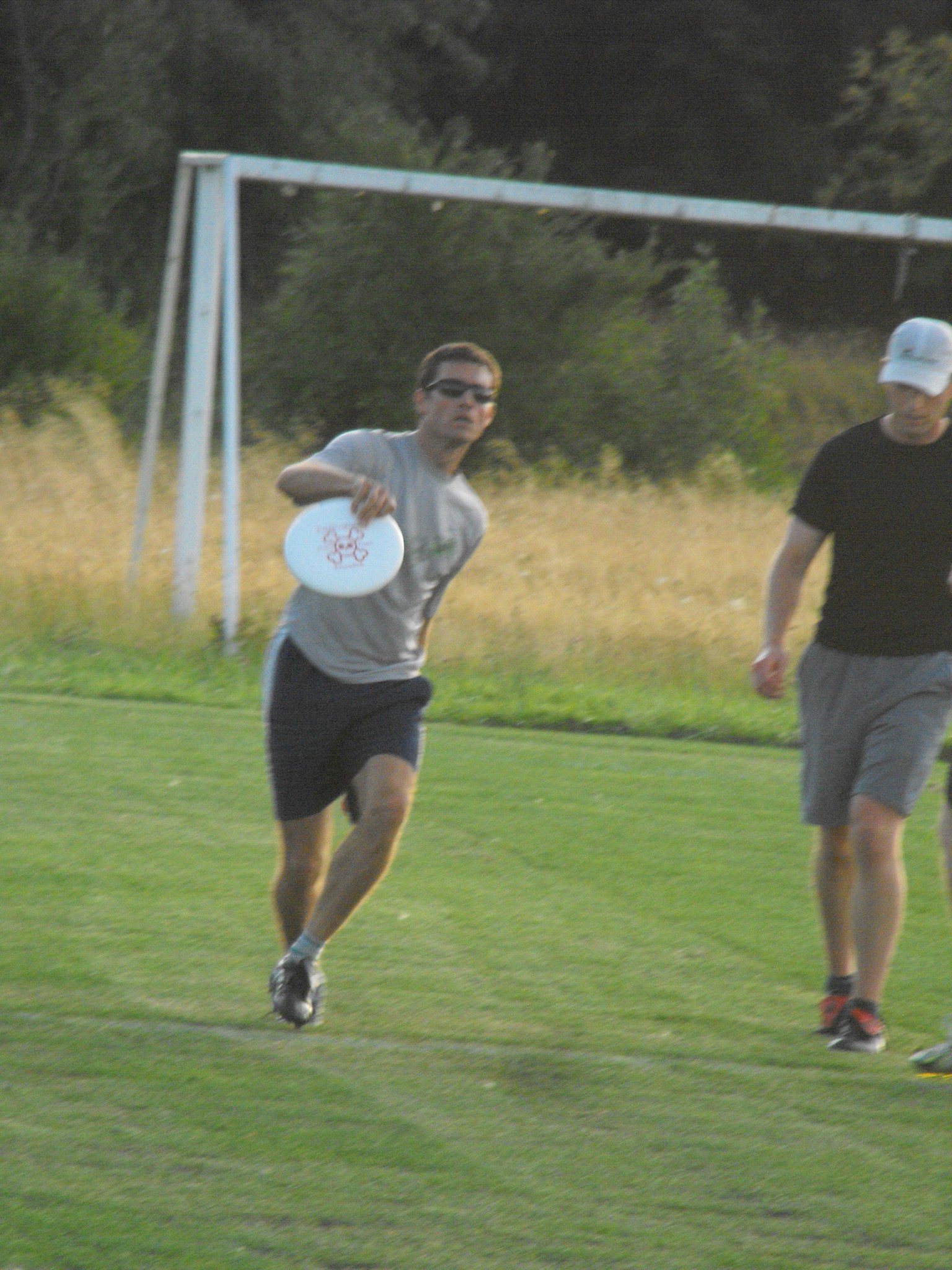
[827,998,887,1056]
[270,955,315,1025]
[813,972,853,1034]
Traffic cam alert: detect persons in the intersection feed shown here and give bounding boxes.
[752,318,952,1056]
[263,340,502,1028]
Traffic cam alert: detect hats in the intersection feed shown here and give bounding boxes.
[878,318,952,395]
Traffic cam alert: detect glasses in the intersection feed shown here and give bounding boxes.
[424,378,497,403]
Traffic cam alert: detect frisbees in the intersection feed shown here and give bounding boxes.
[283,495,407,599]
